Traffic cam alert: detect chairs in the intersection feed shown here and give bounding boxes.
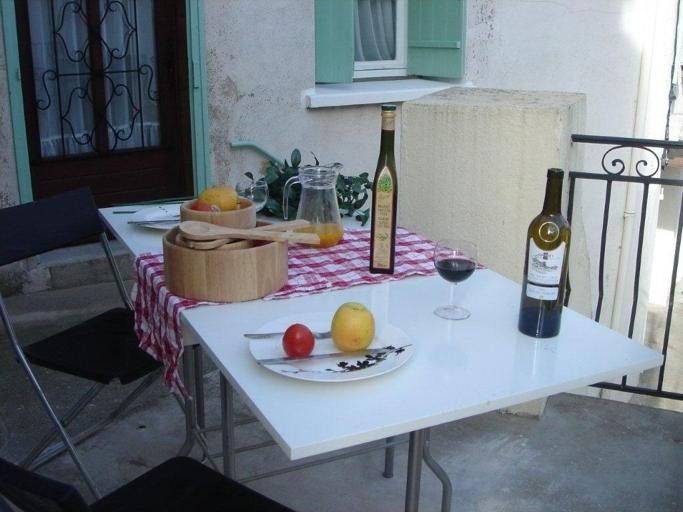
[3,189,285,509]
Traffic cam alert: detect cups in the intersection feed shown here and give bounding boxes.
[431,240,478,322]
[280,164,345,249]
[235,180,269,213]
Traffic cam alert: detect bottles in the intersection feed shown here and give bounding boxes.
[516,167,572,338]
[368,103,401,276]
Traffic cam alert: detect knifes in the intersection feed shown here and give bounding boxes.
[257,345,411,366]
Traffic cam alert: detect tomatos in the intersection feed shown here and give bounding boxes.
[192,201,208,211]
[282,322,316,357]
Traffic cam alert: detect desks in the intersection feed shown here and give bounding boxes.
[96,195,665,512]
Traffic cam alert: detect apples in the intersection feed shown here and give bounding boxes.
[330,302,375,352]
[199,187,241,211]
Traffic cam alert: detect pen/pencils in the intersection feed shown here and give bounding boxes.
[113,211,136,214]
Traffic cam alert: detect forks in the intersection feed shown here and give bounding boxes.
[244,329,330,339]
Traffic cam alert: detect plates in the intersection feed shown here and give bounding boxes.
[248,310,414,382]
[128,202,183,231]
